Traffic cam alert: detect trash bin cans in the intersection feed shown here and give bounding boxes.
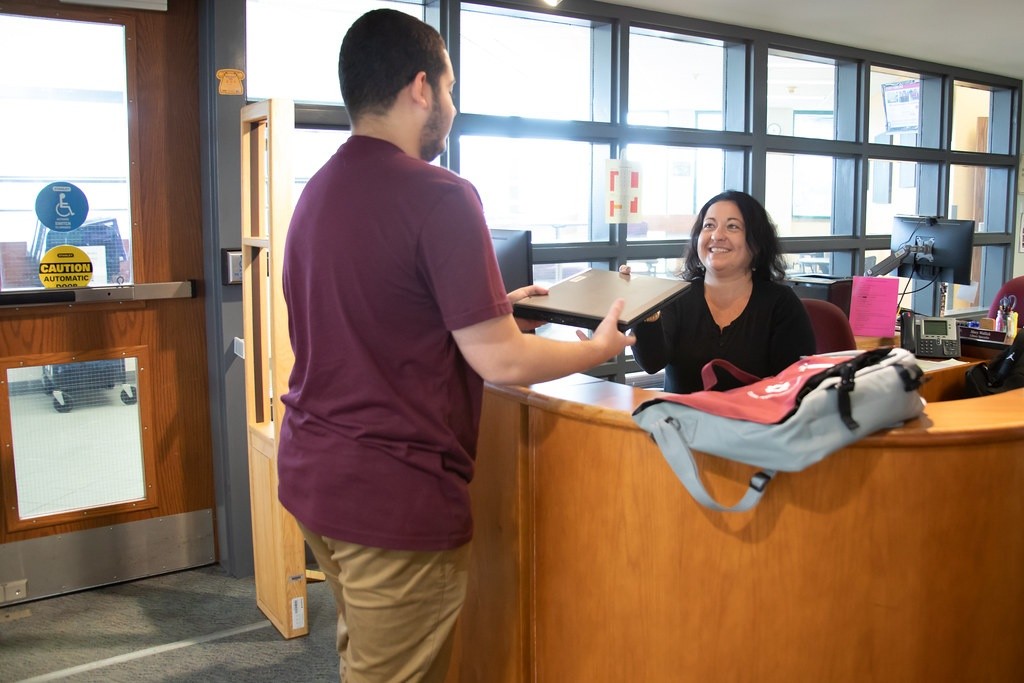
[41,218,140,413]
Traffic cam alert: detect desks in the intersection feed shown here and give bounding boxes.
[798,258,830,275]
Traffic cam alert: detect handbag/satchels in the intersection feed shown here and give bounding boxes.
[631,346,929,512]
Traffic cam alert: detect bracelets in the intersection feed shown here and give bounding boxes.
[644,311,660,322]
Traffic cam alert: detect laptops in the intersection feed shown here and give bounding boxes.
[512,266,691,331]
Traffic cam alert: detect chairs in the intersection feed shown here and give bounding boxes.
[865,255,877,272]
[801,297,857,356]
[963,329,1024,398]
[987,275,1024,327]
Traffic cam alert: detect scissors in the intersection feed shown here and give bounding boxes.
[999,294,1017,311]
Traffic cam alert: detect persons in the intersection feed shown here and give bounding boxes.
[277,8,638,683]
[618,190,816,395]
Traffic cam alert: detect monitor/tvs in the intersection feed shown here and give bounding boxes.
[488,229,536,336]
[891,215,975,286]
[778,273,853,323]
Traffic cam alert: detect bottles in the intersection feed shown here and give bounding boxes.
[996,310,1015,340]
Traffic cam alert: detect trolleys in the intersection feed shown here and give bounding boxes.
[30,217,137,413]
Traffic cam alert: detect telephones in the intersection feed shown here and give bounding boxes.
[901,311,960,359]
